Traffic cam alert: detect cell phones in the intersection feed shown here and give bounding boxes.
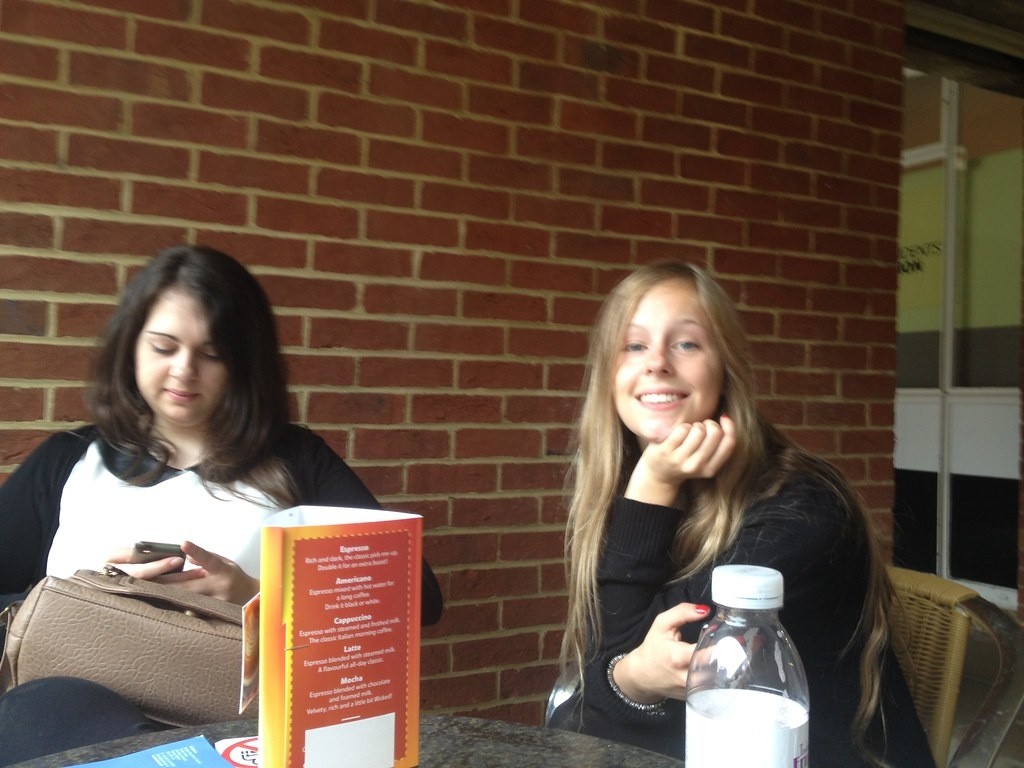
[129,541,186,578]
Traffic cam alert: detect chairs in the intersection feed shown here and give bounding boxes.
[884,567,1024,768]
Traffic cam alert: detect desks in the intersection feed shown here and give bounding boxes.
[0,716,685,768]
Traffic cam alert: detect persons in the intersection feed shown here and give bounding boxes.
[543,261,937,768]
[0,239,443,768]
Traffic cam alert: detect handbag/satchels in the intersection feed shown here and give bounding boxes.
[1,562,261,731]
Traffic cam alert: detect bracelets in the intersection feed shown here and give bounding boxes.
[607,652,672,712]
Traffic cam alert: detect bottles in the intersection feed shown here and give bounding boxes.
[684,564,809,767]
